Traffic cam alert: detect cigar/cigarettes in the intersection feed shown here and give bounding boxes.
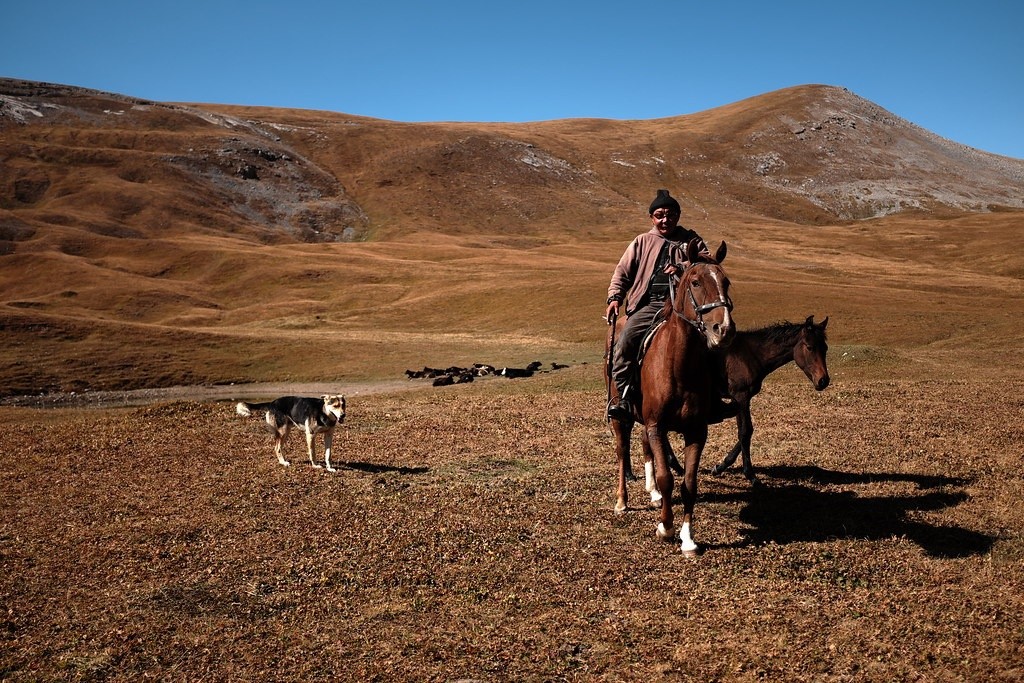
[602,316,613,323]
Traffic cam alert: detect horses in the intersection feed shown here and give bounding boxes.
[602,238,831,557]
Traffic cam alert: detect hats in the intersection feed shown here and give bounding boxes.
[649,190,680,214]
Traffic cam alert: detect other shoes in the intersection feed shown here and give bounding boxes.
[608,400,633,424]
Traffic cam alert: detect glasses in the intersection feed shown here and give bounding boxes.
[654,213,677,219]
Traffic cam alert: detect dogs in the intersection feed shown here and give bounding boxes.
[236,393,346,473]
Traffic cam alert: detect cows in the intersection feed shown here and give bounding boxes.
[404,360,570,386]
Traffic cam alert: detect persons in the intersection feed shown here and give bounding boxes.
[605,189,710,418]
